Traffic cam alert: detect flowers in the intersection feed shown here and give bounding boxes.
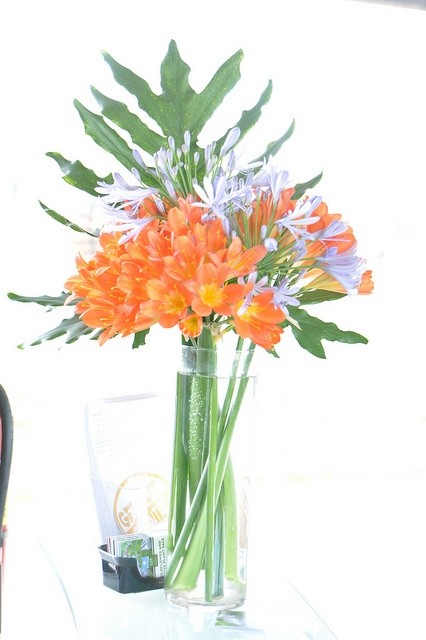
[5,38,375,598]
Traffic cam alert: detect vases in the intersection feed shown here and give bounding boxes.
[162,340,255,610]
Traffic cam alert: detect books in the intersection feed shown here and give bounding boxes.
[105,531,174,578]
